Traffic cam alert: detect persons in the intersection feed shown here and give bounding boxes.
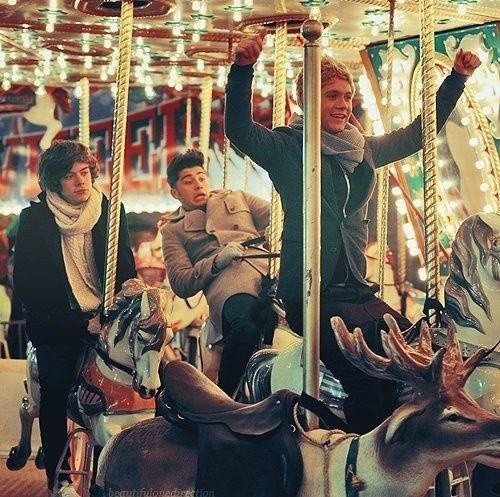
[224,26,481,435]
[11,140,138,497]
[159,148,272,400]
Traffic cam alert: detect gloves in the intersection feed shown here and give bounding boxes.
[211,241,246,271]
